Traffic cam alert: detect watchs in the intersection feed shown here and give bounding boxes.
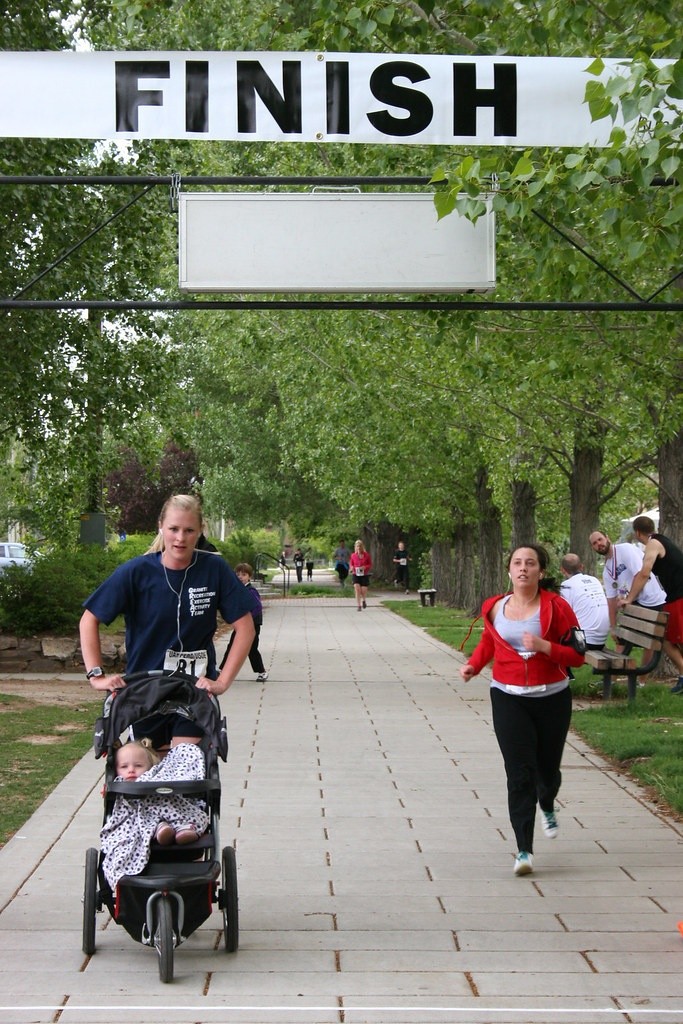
[86,667,104,679]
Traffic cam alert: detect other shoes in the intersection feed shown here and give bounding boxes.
[358,606,361,611]
[394,580,399,588]
[405,589,410,594]
[363,601,366,608]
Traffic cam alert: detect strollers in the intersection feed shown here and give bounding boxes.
[80,671,238,983]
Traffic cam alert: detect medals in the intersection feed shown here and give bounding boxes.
[612,582,618,588]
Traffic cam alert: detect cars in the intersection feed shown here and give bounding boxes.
[0,542,47,578]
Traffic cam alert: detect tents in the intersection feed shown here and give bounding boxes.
[621,507,659,543]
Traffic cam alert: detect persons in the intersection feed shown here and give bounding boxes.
[559,554,610,651]
[460,545,585,875]
[616,516,683,694]
[216,563,270,681]
[590,531,666,688]
[349,540,372,612]
[393,541,413,595]
[303,547,314,582]
[293,549,304,583]
[332,539,351,588]
[114,741,199,844]
[79,494,261,759]
[279,551,286,570]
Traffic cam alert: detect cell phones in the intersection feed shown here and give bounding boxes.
[575,630,586,650]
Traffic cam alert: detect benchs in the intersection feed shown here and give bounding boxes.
[417,588,438,607]
[585,604,670,709]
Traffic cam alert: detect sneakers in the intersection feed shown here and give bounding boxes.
[671,674,683,694]
[155,821,175,845]
[541,809,558,839]
[175,821,198,845]
[513,850,533,874]
[257,671,269,682]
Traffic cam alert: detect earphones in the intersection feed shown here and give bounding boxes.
[508,573,512,578]
[539,572,543,578]
[160,529,163,535]
[198,532,201,538]
